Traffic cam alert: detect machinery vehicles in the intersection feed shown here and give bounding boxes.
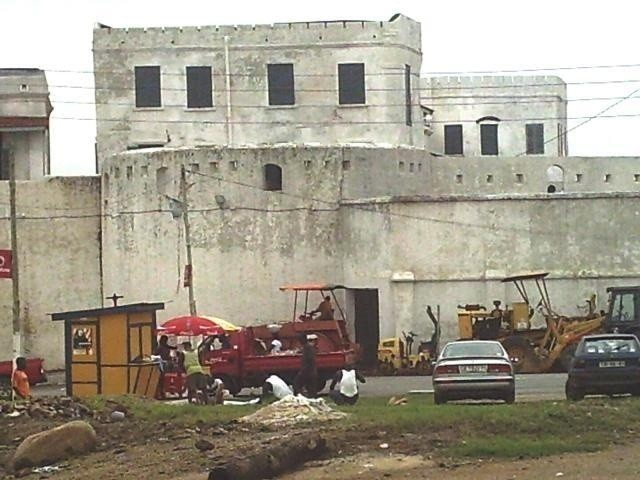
[378,330,440,375]
[456,273,640,374]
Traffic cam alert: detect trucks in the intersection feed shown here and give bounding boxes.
[185,328,361,395]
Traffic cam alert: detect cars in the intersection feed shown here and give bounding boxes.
[567,334,639,400]
[430,341,519,404]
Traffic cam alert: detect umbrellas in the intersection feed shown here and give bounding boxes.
[151,314,247,342]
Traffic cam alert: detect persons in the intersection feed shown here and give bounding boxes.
[329,361,366,407]
[256,370,293,405]
[11,357,32,402]
[291,332,316,398]
[153,334,178,400]
[311,295,331,321]
[266,332,282,354]
[177,342,224,406]
